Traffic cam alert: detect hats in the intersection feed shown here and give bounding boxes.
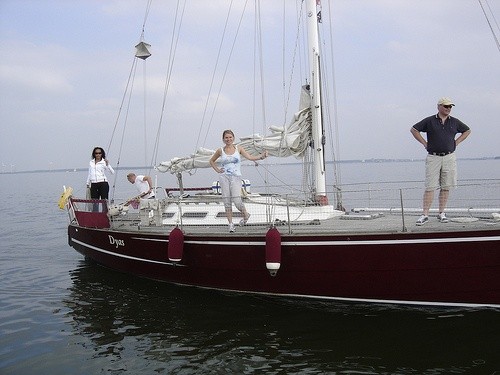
[437,97,455,106]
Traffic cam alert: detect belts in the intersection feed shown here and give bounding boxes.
[429,151,451,156]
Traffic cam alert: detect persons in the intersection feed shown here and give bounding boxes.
[127,173,155,198]
[410,97,471,226]
[86,147,115,214]
[210,130,268,233]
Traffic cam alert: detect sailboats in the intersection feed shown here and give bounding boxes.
[57,0,500,340]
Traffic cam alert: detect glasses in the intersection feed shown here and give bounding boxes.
[95,152,102,154]
[441,105,452,108]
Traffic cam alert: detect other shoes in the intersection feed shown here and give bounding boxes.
[228,224,237,232]
[239,212,251,226]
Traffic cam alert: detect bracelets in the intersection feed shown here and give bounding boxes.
[259,154,264,160]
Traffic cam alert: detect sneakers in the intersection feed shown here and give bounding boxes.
[436,212,448,223]
[416,214,428,225]
